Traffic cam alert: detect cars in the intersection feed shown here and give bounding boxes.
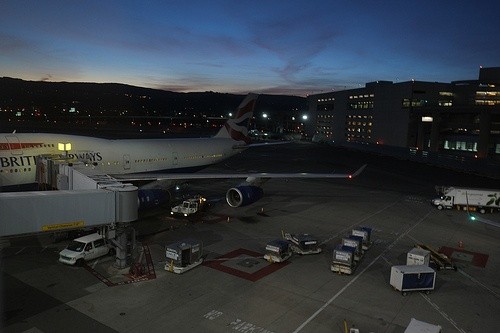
[171,202,198,217]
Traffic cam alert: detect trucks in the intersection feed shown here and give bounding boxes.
[432,185,500,214]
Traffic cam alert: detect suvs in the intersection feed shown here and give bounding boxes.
[58,234,118,267]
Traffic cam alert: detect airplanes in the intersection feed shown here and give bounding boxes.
[1,91,370,209]
